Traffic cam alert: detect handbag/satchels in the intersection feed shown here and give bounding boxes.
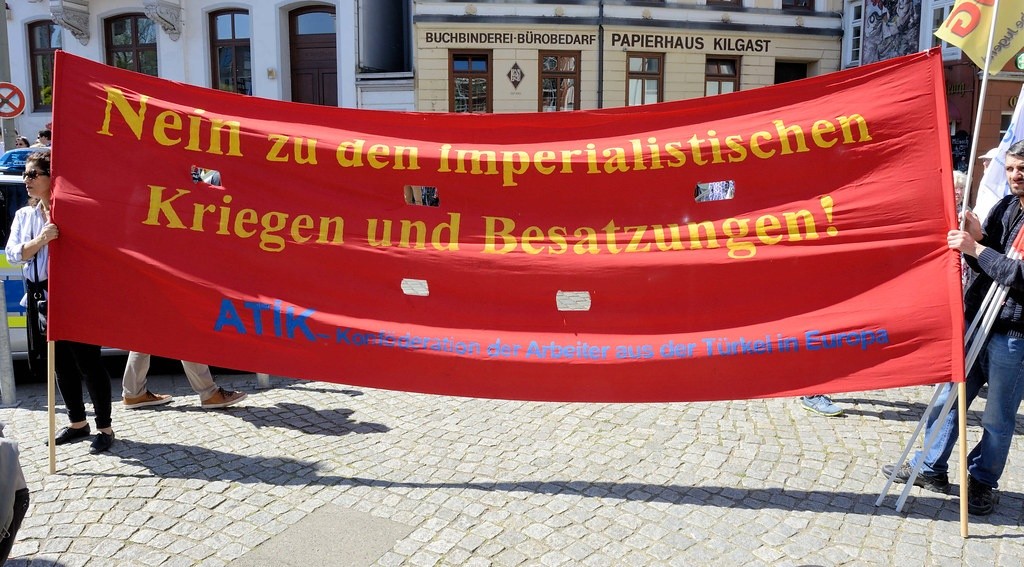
[37,300,48,334]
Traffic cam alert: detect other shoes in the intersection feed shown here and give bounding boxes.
[45,423,90,446]
[89,430,114,454]
[802,393,843,416]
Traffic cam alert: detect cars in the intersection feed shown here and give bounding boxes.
[0,147,163,358]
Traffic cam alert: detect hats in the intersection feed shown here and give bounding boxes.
[978,147,998,159]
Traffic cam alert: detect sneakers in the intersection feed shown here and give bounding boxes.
[123,390,172,409]
[882,461,951,494]
[967,473,995,516]
[201,385,247,408]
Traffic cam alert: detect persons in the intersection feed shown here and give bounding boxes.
[882,140,1024,516]
[0,121,52,151]
[5,152,114,454]
[0,424,30,567]
[801,395,843,416]
[121,350,247,408]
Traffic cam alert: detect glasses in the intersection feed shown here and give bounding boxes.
[38,137,40,139]
[22,170,49,180]
[16,143,25,146]
